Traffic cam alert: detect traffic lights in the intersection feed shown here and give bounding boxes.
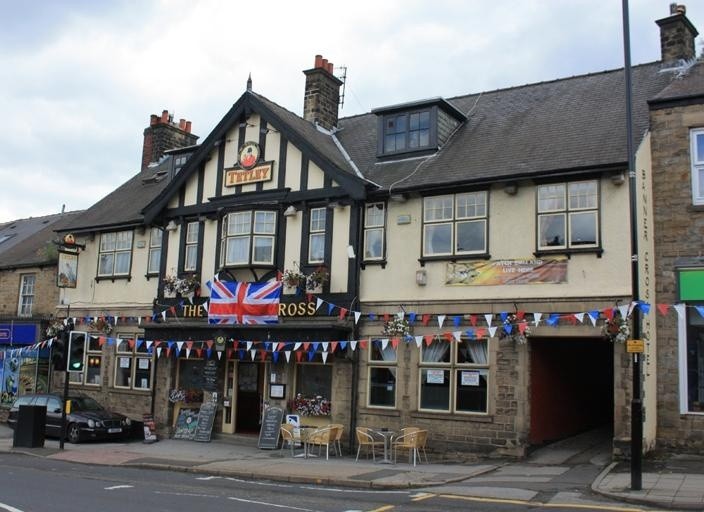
[66,331,88,373]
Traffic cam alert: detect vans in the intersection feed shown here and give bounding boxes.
[6,392,131,443]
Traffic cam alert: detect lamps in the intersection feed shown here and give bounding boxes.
[165,220,178,232]
[415,269,428,286]
[283,204,297,218]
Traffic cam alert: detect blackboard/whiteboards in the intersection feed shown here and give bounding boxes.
[256,405,286,449]
[171,407,200,441]
[203,350,224,391]
[192,401,218,442]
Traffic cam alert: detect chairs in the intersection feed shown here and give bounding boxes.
[279,421,429,468]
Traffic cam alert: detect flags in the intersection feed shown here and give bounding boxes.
[208,280,282,324]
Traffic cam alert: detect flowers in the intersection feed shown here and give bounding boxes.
[167,387,202,403]
[87,314,112,334]
[499,314,532,345]
[282,267,330,289]
[380,316,414,343]
[600,314,631,345]
[293,392,331,416]
[163,270,200,298]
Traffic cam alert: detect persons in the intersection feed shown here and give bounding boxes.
[241,147,255,167]
